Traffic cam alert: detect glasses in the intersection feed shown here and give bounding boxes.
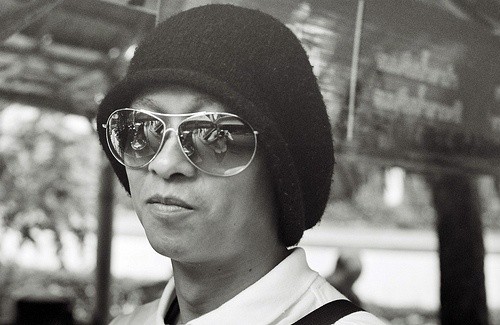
[102,107,259,177]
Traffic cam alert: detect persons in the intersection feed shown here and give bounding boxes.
[96,3,386,325]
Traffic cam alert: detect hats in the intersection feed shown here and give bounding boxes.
[95,4,334,246]
